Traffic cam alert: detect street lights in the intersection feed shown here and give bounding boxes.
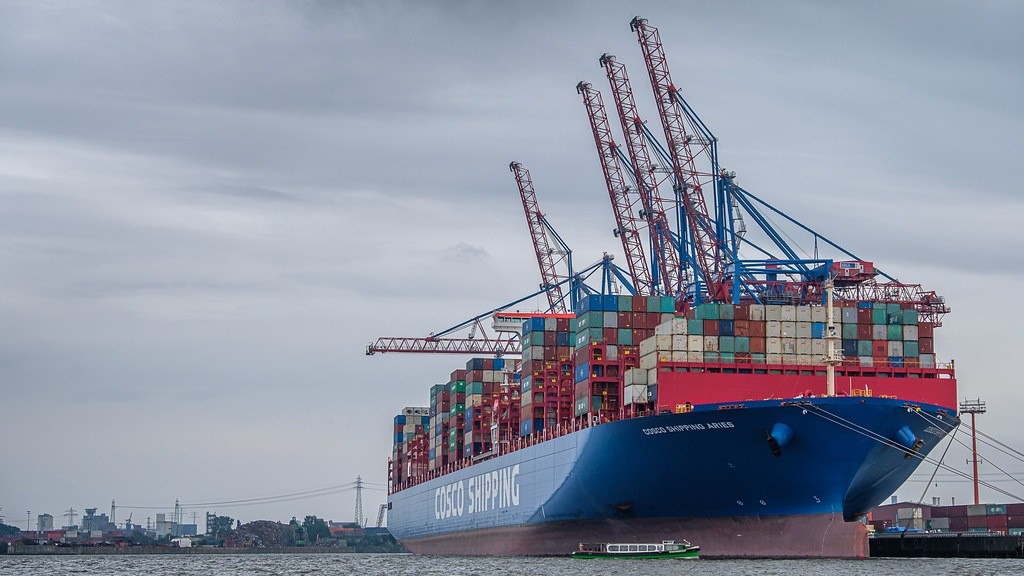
[957,394,987,505]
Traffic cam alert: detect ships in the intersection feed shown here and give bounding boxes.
[365,17,962,560]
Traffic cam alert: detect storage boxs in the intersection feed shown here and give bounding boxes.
[390,292,935,489]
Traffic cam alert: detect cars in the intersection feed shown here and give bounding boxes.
[570,539,700,562]
